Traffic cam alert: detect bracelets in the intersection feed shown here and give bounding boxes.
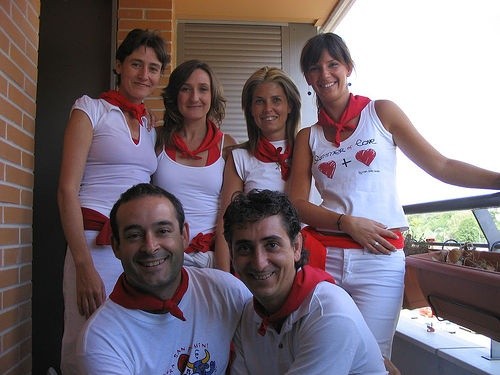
[337,214,344,231]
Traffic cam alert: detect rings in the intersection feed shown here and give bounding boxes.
[374,242,378,247]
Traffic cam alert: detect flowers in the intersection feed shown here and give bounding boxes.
[401,230,436,257]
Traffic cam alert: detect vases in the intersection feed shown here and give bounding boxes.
[402,265,431,310]
[405,251,500,342]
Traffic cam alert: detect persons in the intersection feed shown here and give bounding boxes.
[151,59,237,269]
[57,30,170,375]
[76,184,402,375]
[216,66,316,282]
[221,186,389,375]
[289,30,500,362]
